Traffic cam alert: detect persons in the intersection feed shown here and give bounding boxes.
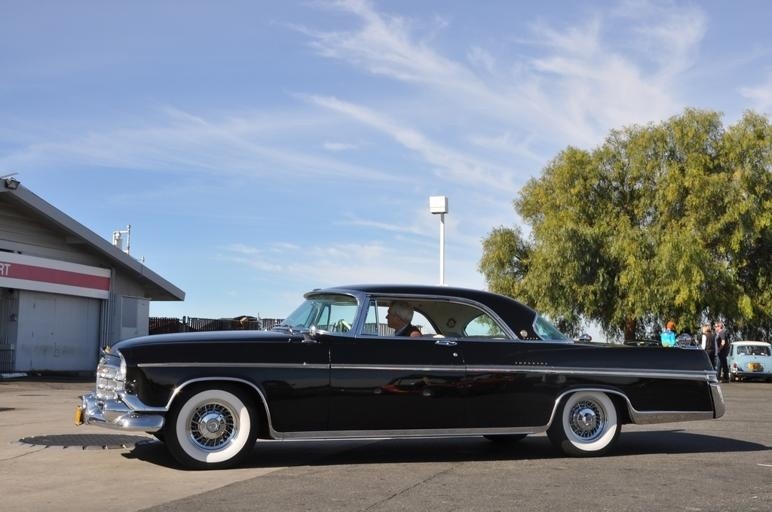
[701,325,714,365]
[385,301,422,337]
[714,321,731,384]
[660,320,676,348]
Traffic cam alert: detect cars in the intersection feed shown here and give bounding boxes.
[728,341,772,383]
[74,284,727,471]
